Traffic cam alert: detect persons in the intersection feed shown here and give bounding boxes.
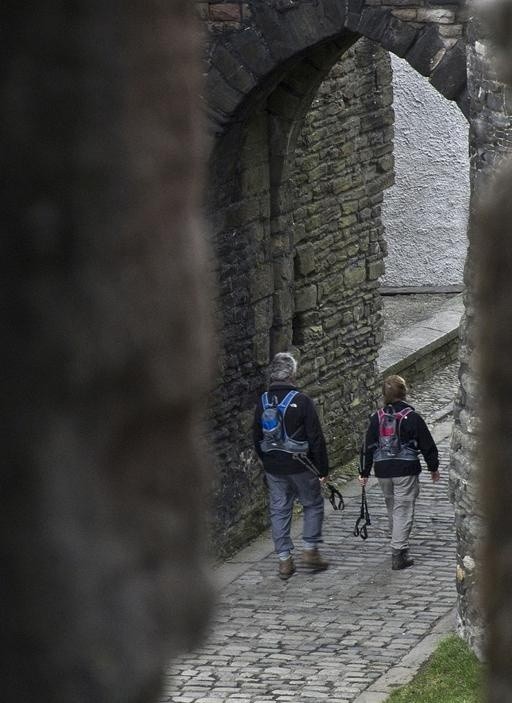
[253,351,329,580]
[359,375,439,570]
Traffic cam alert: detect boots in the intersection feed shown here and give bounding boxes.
[302,548,329,573]
[391,548,414,570]
[279,555,296,579]
